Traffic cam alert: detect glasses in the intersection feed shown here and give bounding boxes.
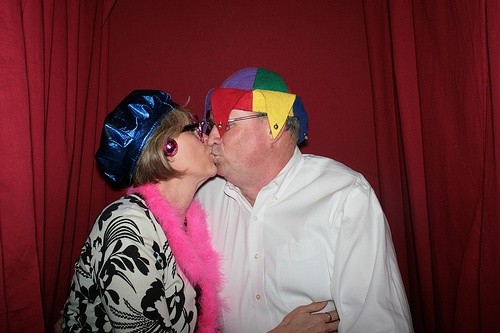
[179,120,203,142]
[203,111,268,137]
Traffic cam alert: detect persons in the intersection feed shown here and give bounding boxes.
[54,70,411,333]
[58,90,340,333]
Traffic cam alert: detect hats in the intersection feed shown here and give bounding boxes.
[206,67,312,135]
[96,88,182,190]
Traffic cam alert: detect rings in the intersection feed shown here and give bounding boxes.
[325,312,333,322]
